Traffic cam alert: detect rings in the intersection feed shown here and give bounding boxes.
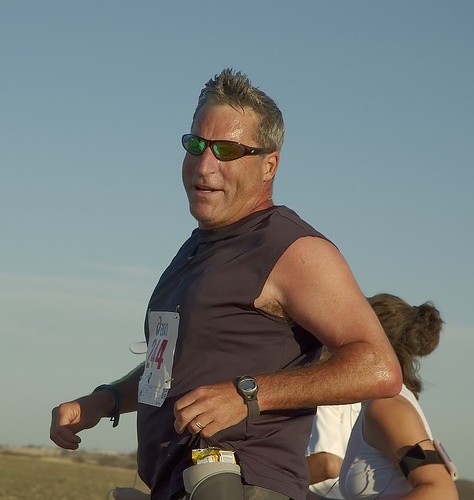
[193,418,204,429]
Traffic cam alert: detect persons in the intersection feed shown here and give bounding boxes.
[305,341,361,500]
[339,294,458,500]
[49,69,402,500]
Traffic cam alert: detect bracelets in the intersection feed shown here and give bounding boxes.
[92,384,120,428]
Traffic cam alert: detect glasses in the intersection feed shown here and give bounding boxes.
[181,134,276,162]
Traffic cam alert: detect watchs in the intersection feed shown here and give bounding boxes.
[233,375,260,418]
